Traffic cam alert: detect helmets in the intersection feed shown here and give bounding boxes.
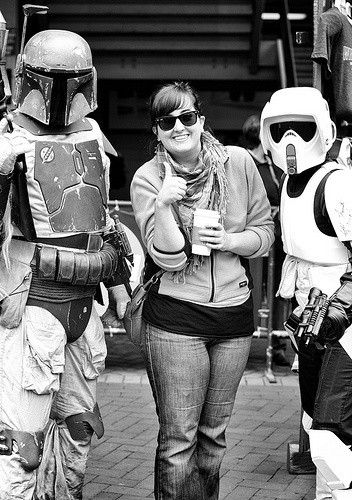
[258,83,337,177]
[11,28,103,125]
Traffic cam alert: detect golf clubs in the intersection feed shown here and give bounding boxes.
[20,2,49,56]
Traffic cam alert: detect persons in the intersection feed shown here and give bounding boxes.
[0,29,132,500]
[244,87,352,500]
[129,81,276,500]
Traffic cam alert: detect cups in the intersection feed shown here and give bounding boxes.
[190,208,220,257]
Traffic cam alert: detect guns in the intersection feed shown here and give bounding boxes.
[298,287,331,350]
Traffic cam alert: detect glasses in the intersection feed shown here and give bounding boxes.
[152,109,203,131]
